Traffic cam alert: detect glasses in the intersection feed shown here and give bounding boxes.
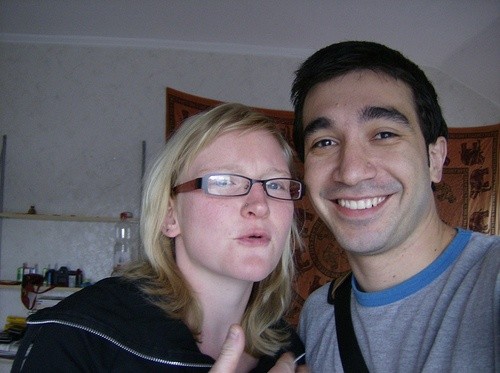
[170,173,305,201]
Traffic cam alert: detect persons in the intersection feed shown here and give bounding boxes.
[292,40,498,373]
[11,104,311,373]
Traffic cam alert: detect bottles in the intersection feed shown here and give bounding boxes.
[113,212,139,267]
